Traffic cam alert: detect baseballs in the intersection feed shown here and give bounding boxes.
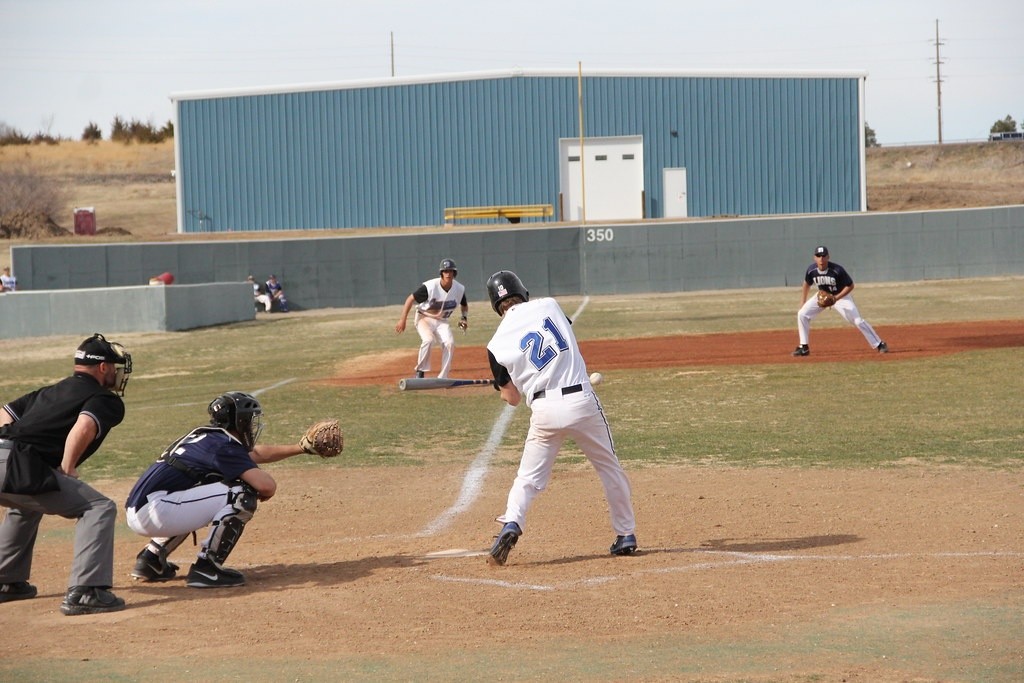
[589,373,602,385]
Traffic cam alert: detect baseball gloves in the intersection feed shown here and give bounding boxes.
[298,418,344,457]
[458,321,468,336]
[817,289,837,307]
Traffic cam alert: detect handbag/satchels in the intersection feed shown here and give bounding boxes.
[1,439,61,496]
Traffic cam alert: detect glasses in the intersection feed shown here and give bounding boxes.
[816,255,826,257]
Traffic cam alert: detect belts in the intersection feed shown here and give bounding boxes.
[534,384,582,399]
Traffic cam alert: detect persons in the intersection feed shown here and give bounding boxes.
[396,258,468,378]
[792,246,888,356]
[0,266,16,291]
[0,333,133,615]
[124,392,344,587]
[487,271,638,567]
[247,274,289,313]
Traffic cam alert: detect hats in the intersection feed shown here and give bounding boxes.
[814,246,828,256]
[75,336,126,366]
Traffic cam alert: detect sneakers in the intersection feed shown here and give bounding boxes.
[490,523,520,566]
[60,587,125,615]
[187,563,245,587]
[0,582,37,602]
[610,535,637,554]
[131,549,180,582]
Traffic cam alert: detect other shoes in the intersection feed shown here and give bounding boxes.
[878,342,888,353]
[793,346,809,356]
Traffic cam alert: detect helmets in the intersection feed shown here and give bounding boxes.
[208,391,263,450]
[439,259,457,278]
[486,270,530,317]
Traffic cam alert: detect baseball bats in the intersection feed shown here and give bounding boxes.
[398,378,495,391]
